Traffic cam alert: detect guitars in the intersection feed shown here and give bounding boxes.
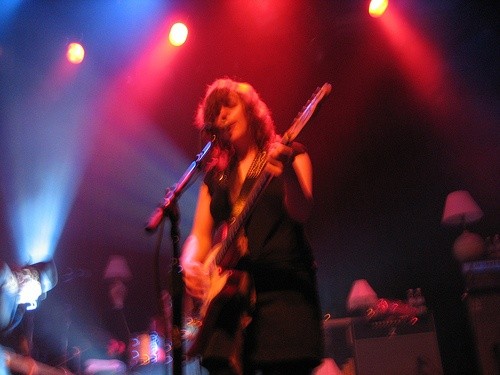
[192,82,332,328]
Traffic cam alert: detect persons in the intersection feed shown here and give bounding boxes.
[176,81,327,373]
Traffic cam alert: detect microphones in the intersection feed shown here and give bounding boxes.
[204,122,232,141]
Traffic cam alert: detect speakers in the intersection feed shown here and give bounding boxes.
[351,315,443,374]
[430,256,499,374]
